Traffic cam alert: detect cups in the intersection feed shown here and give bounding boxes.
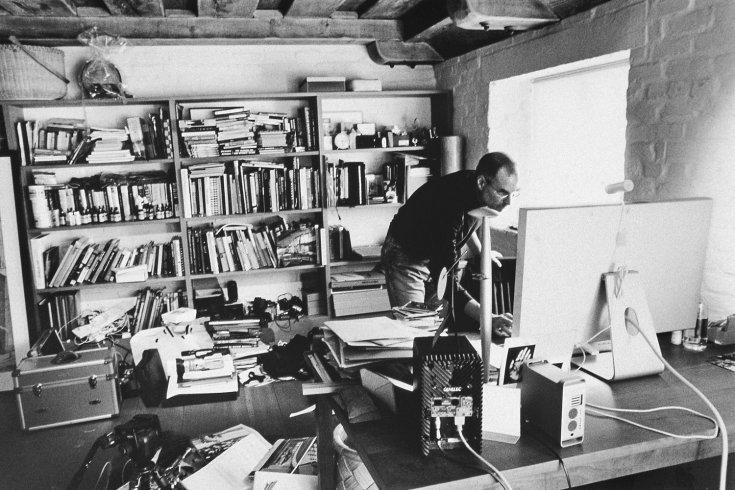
[332,230,351,260]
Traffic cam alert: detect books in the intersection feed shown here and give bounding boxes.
[322,309,452,371]
[336,162,366,208]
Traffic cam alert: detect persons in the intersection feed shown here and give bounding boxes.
[381,153,521,337]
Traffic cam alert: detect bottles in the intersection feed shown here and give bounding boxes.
[350,127,362,149]
[669,301,709,353]
[324,134,333,150]
[427,127,437,138]
[47,204,172,225]
[376,130,394,148]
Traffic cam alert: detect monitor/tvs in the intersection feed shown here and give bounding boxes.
[513,196,713,382]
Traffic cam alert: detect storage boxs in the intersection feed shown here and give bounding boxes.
[299,77,345,92]
[14,346,126,436]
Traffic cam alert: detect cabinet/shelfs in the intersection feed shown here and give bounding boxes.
[318,92,450,325]
[0,97,192,355]
[167,93,331,339]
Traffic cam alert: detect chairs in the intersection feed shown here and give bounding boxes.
[443,254,516,331]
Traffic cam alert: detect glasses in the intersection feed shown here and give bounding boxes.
[488,180,522,199]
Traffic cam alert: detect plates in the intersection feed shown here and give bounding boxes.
[334,133,350,149]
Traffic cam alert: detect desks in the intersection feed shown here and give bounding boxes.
[304,315,734,490]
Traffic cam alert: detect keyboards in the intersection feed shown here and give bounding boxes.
[573,341,611,355]
